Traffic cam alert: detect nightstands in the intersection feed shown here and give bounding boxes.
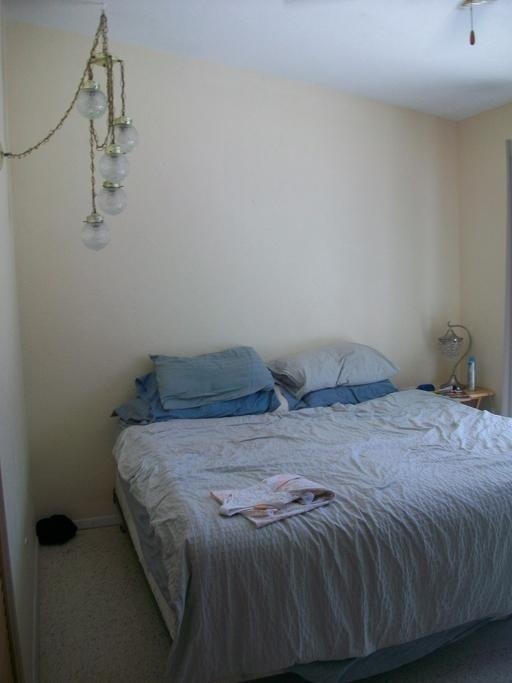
[433,383,495,410]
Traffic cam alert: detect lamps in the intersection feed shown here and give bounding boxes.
[439,321,472,392]
[0,0,138,251]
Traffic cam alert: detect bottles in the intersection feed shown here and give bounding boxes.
[467,357,477,391]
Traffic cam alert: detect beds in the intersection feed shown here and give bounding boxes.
[110,337,511,683]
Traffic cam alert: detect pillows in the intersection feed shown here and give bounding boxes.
[152,348,277,411]
[277,381,397,409]
[114,405,144,429]
[271,341,396,399]
[135,372,279,417]
